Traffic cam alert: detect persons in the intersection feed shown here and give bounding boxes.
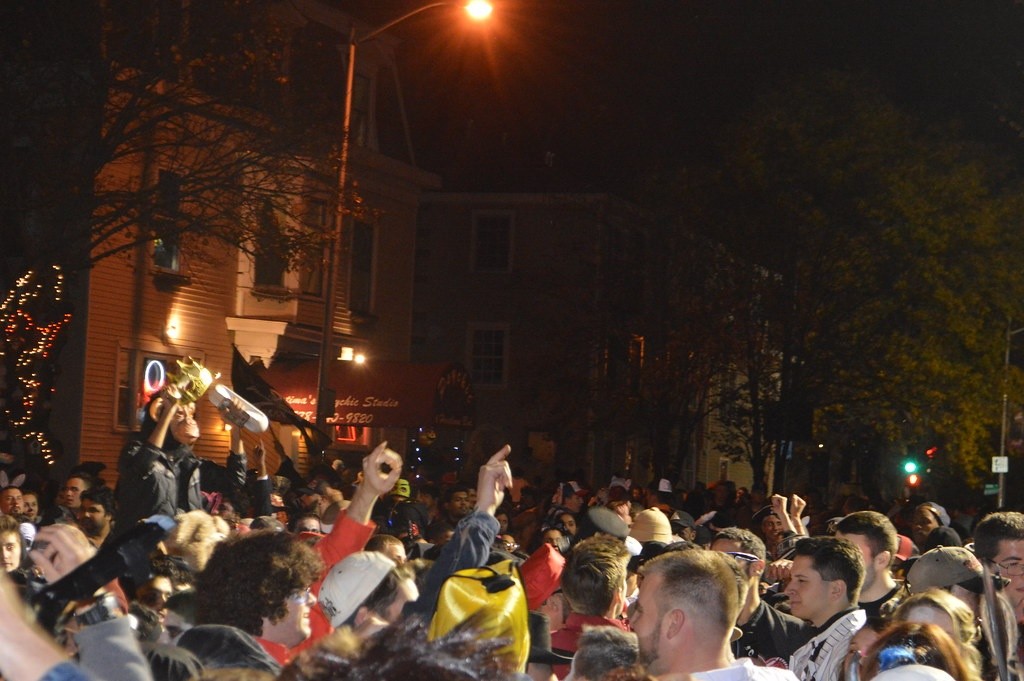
[0,386,1024,681]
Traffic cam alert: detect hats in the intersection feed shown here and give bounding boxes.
[135,515,177,532]
[582,506,630,539]
[557,481,590,497]
[776,534,809,560]
[669,510,696,529]
[751,505,790,521]
[317,550,396,627]
[525,610,576,664]
[915,502,951,526]
[630,507,673,545]
[906,544,1011,594]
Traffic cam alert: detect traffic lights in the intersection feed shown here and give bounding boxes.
[905,459,919,489]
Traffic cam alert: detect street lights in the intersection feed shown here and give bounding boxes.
[310,1,496,464]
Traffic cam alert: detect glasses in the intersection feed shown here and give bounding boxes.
[504,542,518,548]
[726,551,759,562]
[983,556,1024,576]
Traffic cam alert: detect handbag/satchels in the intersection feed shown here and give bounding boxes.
[427,559,530,672]
